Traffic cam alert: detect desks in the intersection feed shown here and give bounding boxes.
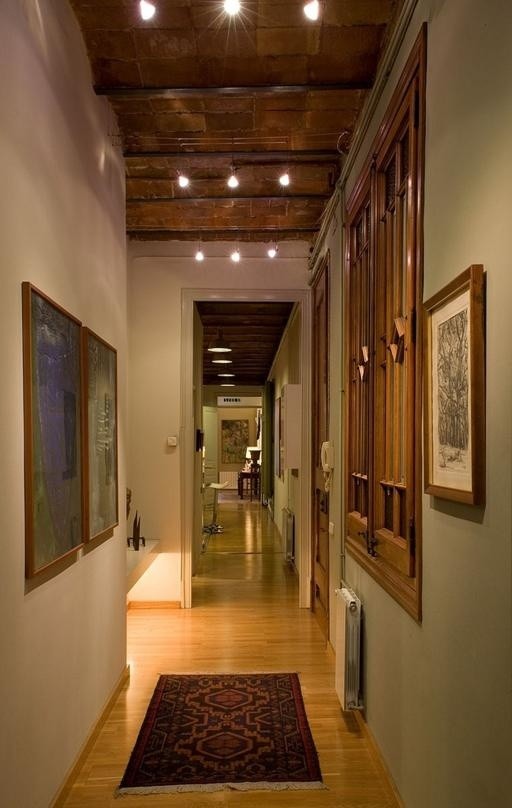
[239,472,260,500]
[127,538,162,596]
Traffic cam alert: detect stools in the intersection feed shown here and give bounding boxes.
[202,481,230,534]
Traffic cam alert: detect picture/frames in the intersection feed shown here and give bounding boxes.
[423,264,486,507]
[24,282,118,580]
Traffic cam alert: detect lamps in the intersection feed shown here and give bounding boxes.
[207,328,232,353]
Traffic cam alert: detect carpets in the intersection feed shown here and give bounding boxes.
[113,672,330,800]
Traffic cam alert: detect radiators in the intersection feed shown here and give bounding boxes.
[334,588,365,713]
[281,505,294,561]
